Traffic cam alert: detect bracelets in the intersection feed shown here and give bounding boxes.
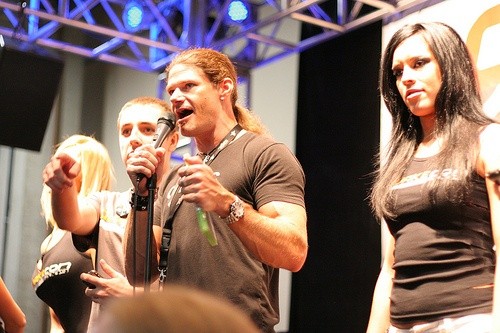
[129,193,150,211]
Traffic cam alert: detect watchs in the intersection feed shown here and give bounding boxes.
[219,195,246,225]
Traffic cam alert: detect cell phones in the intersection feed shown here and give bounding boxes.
[83,271,104,289]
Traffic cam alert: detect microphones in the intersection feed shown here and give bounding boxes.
[137,111,176,183]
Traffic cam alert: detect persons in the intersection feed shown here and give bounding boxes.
[366,21,500,333]
[30,134,117,333]
[0,276,27,333]
[122,48,308,333]
[42,96,180,333]
